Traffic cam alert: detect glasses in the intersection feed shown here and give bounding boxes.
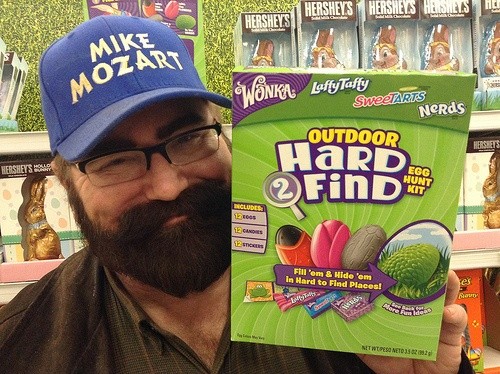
[71,118,221,187]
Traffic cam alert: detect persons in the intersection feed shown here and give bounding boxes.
[0,15,476,373]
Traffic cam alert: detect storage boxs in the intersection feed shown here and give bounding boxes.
[229,67,479,359]
[296,0,359,67]
[463,136,500,230]
[471,0,499,110]
[420,0,483,110]
[359,0,420,69]
[454,267,484,372]
[232,11,294,67]
[0,159,73,260]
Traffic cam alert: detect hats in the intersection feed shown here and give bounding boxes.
[38,16,235,162]
